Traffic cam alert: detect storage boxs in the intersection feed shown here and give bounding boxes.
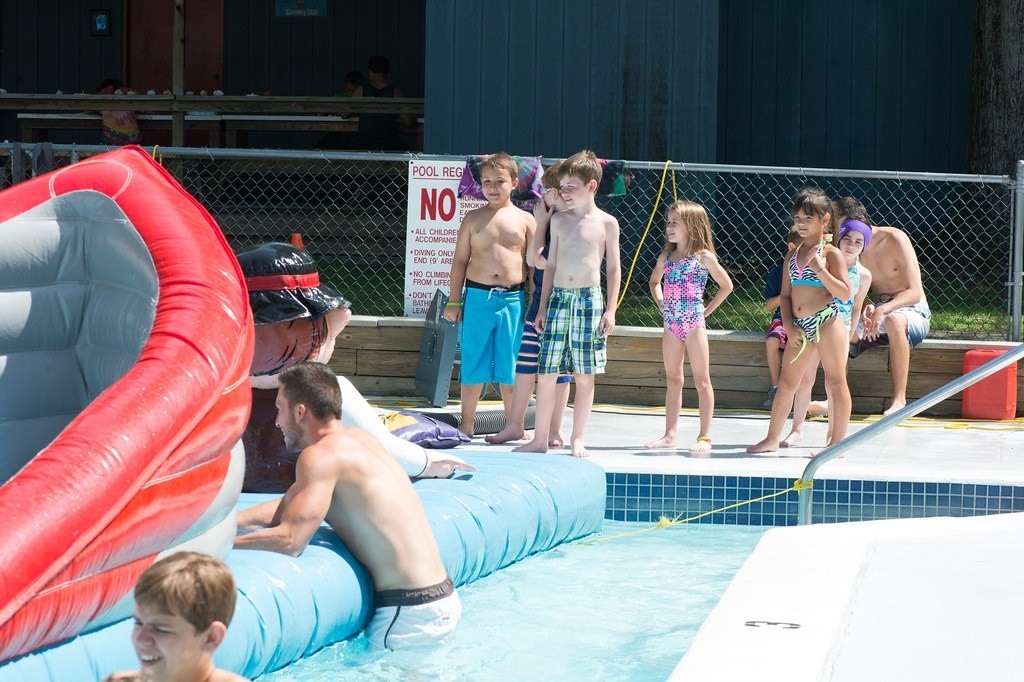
[964,347,1016,419]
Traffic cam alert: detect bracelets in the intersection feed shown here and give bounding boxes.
[447,302,461,306]
[818,268,824,272]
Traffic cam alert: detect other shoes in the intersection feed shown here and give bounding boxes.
[762,386,778,409]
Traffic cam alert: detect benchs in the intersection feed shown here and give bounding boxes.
[346,314,1024,351]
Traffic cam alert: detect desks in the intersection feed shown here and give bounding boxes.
[0,93,423,200]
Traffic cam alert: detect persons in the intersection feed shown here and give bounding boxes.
[234,242,475,494]
[747,189,852,458]
[512,151,621,459]
[444,152,537,441]
[779,211,871,448]
[808,197,931,417]
[763,225,805,409]
[643,200,733,452]
[316,71,364,149]
[107,552,246,682]
[340,56,414,205]
[85,78,146,146]
[233,362,461,645]
[485,158,572,447]
